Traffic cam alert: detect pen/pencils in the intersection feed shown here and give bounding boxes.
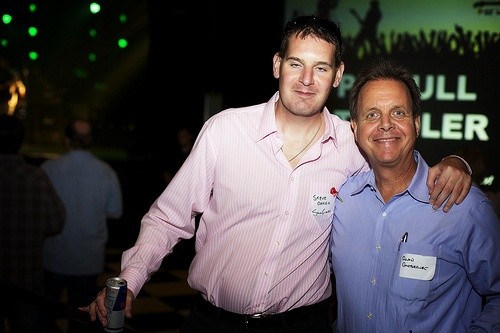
[398,229,408,251]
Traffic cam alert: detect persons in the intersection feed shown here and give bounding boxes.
[26,116,123,333]
[78,14,473,333]
[330,65,500,333]
[0,115,63,333]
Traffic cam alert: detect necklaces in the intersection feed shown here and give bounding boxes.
[280,111,322,163]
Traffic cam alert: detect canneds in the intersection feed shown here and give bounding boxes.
[104,277,127,332]
